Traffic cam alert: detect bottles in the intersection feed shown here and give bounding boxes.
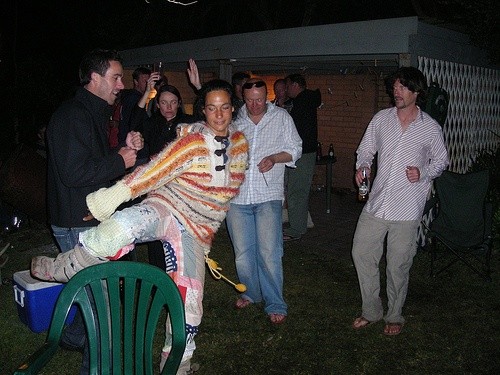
[317,143,321,162]
[358,167,369,201]
[329,144,334,160]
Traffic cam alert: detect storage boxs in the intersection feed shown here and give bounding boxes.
[14,269,78,332]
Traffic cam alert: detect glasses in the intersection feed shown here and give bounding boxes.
[242,81,265,89]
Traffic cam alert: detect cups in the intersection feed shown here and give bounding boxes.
[154,59,166,83]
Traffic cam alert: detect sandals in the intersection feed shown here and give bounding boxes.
[281,230,299,243]
[234,297,251,308]
[351,316,375,329]
[383,323,402,336]
[269,313,284,324]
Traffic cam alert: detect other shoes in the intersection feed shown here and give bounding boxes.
[30,255,58,282]
[282,209,289,224]
[307,212,314,228]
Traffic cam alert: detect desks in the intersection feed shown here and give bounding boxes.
[315,156,338,215]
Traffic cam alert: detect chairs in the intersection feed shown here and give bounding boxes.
[13,261,187,375]
[416,167,496,280]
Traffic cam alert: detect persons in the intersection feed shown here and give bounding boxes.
[31,49,321,375]
[351,66,449,337]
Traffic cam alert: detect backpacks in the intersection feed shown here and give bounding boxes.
[60,310,86,352]
